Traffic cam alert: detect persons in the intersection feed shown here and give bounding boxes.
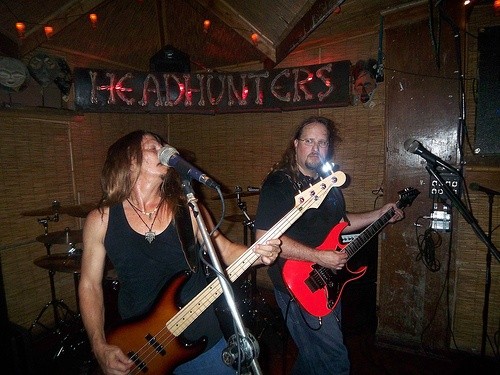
[78,129,281,375]
[255,117,404,375]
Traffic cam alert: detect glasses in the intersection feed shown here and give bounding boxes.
[298,138,329,148]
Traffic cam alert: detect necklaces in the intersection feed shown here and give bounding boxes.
[127,198,164,245]
[131,203,159,220]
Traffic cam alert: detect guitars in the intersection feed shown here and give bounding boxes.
[282,186,419,318]
[106,163,347,375]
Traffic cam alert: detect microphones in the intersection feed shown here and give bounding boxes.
[158,144,219,189]
[404,138,456,173]
[469,183,500,195]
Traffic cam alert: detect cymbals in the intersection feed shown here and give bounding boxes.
[32,252,82,273]
[65,201,99,218]
[36,229,83,244]
[213,191,260,200]
[19,205,66,217]
